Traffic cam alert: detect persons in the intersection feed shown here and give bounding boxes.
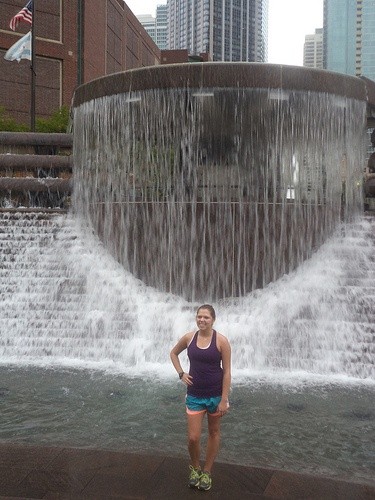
[169,304,231,490]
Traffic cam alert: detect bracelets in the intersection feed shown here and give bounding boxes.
[178,372,184,379]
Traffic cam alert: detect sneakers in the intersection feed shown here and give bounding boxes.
[198,471,212,490]
[188,464,201,487]
